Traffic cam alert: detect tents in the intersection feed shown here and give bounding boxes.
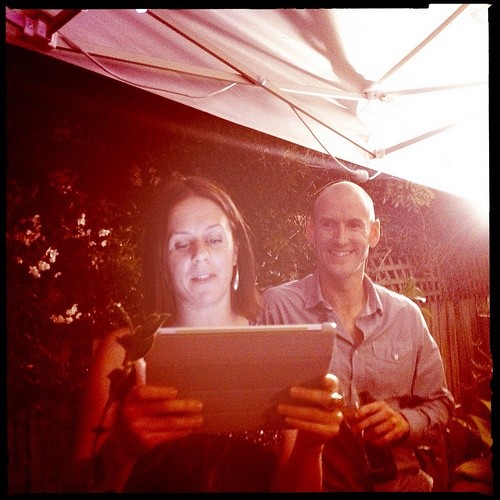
[4,3,490,204]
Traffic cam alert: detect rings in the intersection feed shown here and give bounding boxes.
[327,393,343,412]
[389,417,398,426]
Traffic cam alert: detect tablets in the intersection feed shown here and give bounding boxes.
[132,321,339,430]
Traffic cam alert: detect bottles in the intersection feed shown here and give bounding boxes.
[356,391,396,481]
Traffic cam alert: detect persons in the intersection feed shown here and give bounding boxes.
[246,180,455,494]
[61,172,343,494]
